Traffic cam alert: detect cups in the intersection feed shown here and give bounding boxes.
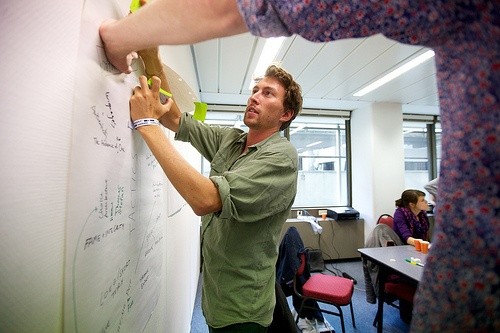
[322,213,326,219]
[414,239,428,254]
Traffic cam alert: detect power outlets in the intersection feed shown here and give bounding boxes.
[297,211,302,216]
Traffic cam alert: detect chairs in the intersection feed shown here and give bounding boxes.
[377,214,394,229]
[285,227,356,333]
[372,224,416,327]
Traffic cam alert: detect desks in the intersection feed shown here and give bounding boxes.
[358,245,429,333]
[280,217,364,262]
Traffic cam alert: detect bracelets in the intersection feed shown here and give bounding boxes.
[133,117,161,129]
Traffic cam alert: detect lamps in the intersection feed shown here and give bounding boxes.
[350,47,435,97]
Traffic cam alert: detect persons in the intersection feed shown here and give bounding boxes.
[423,178,440,213]
[128,0,303,333]
[393,189,430,324]
[98,0,500,333]
